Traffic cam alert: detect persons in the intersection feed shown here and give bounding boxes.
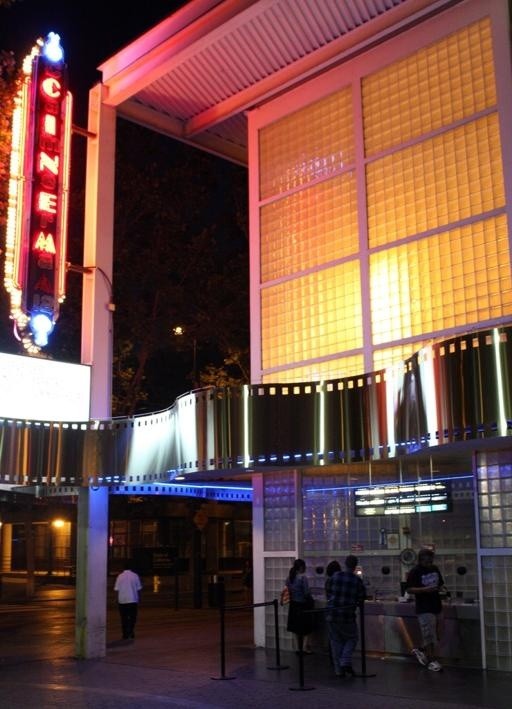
[113,559,145,640]
[285,559,313,656]
[325,555,367,680]
[405,548,446,672]
[323,560,341,664]
[241,560,253,603]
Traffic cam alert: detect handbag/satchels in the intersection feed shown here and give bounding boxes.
[281,574,291,606]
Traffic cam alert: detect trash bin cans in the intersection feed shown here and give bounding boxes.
[207,575,225,608]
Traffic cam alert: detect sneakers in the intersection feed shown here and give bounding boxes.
[410,648,429,665]
[296,648,354,680]
[428,660,442,671]
